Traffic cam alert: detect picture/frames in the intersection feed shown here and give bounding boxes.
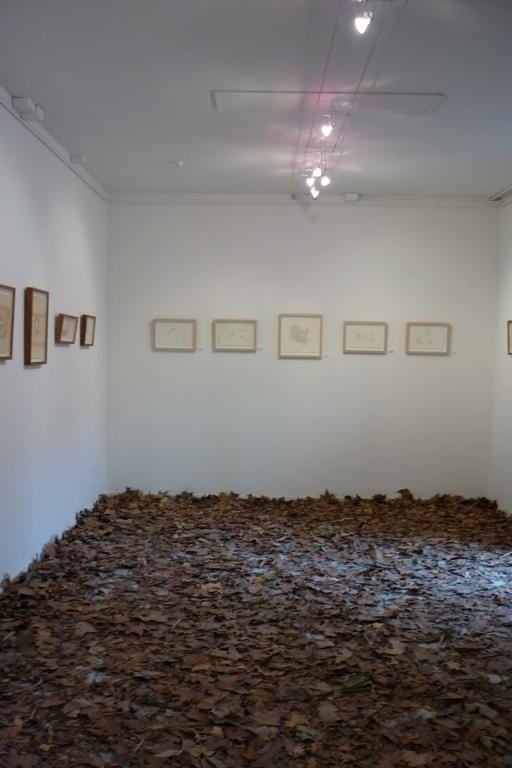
[80,314,96,345]
[212,319,258,353]
[55,311,79,345]
[0,281,16,361]
[403,319,454,356]
[25,285,51,366]
[340,318,388,358]
[150,318,196,353]
[507,319,512,355]
[275,312,323,361]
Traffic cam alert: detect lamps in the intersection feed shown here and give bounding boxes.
[303,1,377,200]
